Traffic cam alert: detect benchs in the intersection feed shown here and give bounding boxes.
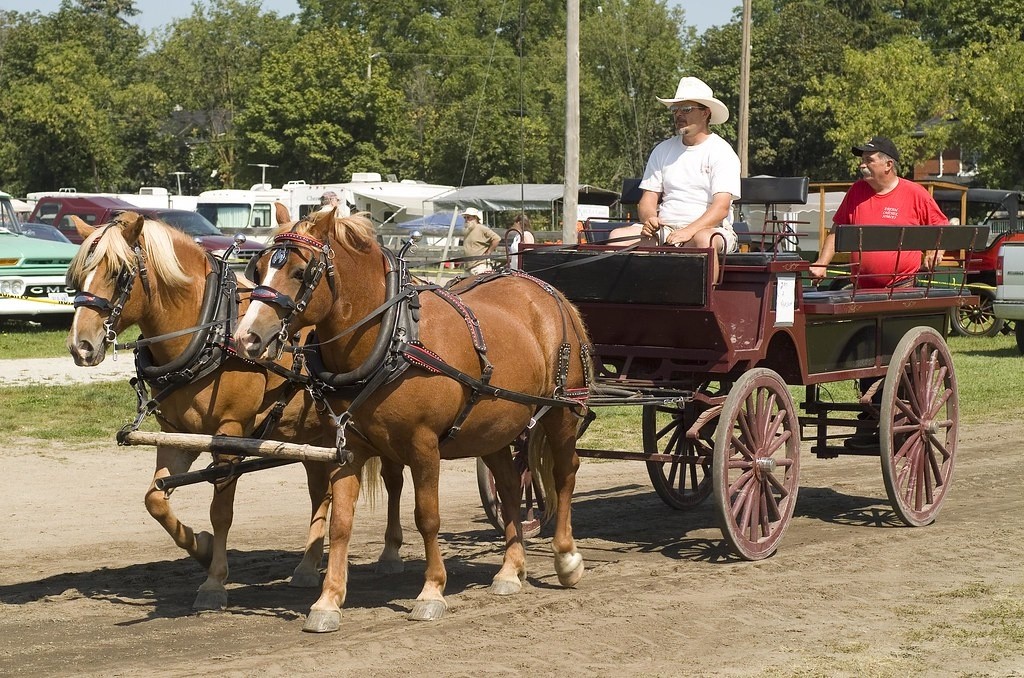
[801,225,991,314]
[586,177,809,274]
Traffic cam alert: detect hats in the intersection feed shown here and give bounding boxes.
[656,76,729,125]
[462,208,481,220]
[852,136,899,162]
[321,191,340,202]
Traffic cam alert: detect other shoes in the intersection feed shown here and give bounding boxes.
[844,432,880,450]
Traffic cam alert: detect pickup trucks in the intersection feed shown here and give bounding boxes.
[39,197,265,263]
[992,241,1024,357]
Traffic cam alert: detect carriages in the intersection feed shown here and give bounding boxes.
[65,172,990,632]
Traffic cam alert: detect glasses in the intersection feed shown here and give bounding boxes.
[670,103,703,114]
[464,216,474,220]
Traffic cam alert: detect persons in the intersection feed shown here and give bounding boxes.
[809,136,950,448]
[511,213,535,271]
[461,208,501,276]
[605,77,742,290]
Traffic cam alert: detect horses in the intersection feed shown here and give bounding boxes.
[64,210,403,610]
[233,202,595,633]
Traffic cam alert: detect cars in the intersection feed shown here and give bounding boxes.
[1,222,74,243]
[0,193,85,318]
